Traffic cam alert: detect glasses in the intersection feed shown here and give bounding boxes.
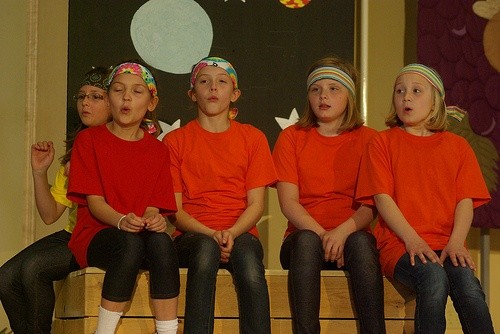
[72,94,104,102]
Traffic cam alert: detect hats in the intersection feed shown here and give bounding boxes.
[77,66,113,90]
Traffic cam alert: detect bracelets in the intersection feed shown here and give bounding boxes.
[117,215,127,230]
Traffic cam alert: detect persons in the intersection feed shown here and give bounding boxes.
[162,55,273,334]
[272,58,386,334]
[65,59,181,334]
[0,65,114,334]
[355,63,496,334]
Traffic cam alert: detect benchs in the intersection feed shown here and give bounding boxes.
[52,268,419,334]
